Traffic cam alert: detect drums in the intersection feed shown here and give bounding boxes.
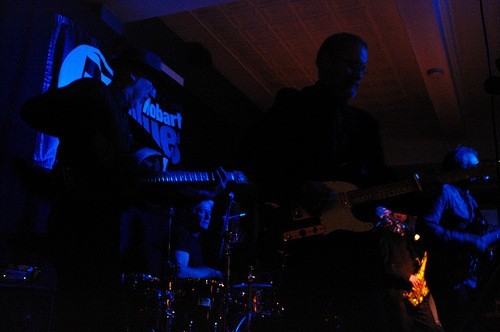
[173,276,230,332]
[232,281,274,319]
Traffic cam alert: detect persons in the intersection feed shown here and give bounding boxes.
[238,32,408,332]
[18,49,171,297]
[415,144,490,332]
[175,198,215,265]
[378,209,442,332]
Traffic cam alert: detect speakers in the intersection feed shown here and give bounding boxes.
[0,283,60,332]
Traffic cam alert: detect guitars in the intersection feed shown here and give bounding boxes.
[62,164,251,208]
[254,161,500,244]
